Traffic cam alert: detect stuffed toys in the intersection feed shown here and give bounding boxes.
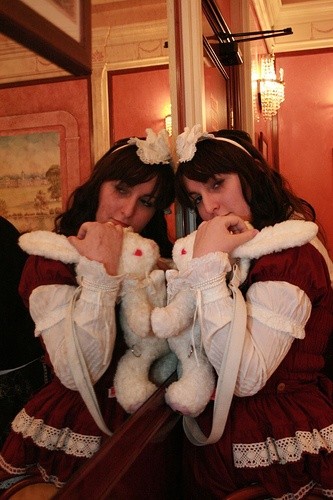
[17,224,179,417]
[150,220,257,420]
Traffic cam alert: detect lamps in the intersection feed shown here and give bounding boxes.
[259,53,292,121]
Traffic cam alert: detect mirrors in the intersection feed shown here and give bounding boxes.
[1,0,212,500]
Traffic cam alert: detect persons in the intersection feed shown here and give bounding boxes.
[0,213,54,453]
[0,126,176,500]
[150,123,332,500]
